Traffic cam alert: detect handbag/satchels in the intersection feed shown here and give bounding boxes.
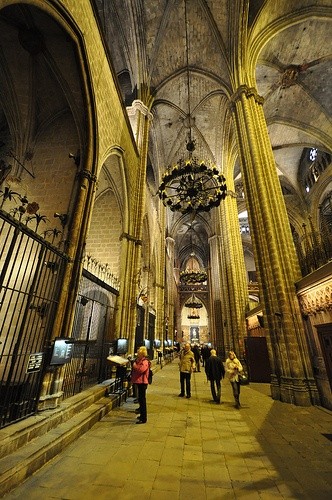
[238,369,249,385]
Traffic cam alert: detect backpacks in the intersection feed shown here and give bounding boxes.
[141,359,153,385]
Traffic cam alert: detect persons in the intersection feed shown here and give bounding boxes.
[205,349,225,404]
[135,407,141,414]
[133,398,139,403]
[224,351,244,409]
[128,347,139,398]
[178,343,195,398]
[178,343,212,373]
[126,346,149,424]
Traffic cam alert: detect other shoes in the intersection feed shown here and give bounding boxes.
[186,394,191,398]
[179,393,185,396]
[134,398,140,414]
[136,420,147,424]
[137,417,141,419]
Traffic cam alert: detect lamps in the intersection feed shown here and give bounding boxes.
[184,292,203,309]
[179,254,208,284]
[157,139,227,214]
[187,309,200,319]
